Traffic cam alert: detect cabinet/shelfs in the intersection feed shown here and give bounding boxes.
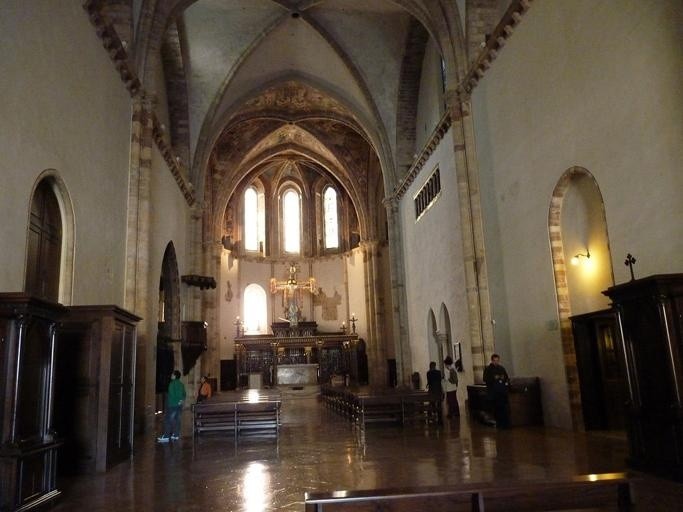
[56,304,144,472]
[0,292,71,512]
[602,272,683,484]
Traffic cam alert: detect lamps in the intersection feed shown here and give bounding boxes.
[569,252,593,265]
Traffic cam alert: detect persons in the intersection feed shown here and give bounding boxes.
[196,374,212,403]
[156,370,187,442]
[441,356,459,417]
[482,353,513,431]
[425,360,444,426]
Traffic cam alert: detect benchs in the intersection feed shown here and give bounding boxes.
[321,386,443,431]
[190,389,282,450]
